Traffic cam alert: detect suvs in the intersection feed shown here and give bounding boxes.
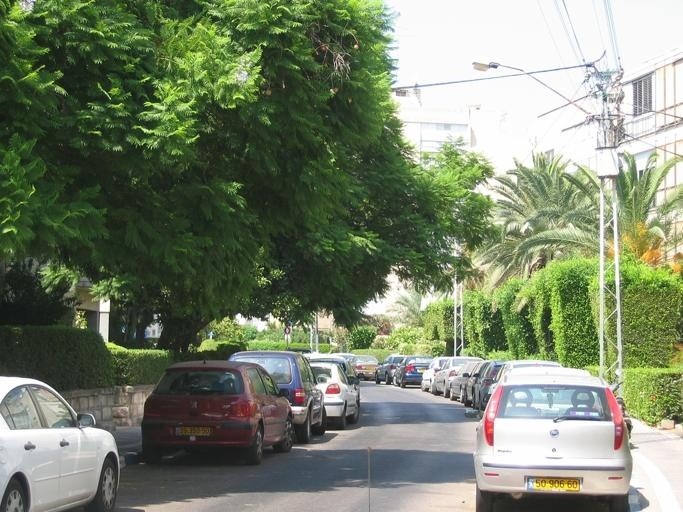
[229,350,327,444]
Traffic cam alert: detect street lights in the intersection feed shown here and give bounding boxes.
[473,60,625,397]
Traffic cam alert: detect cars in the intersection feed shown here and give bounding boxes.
[1,375,120,511]
[474,366,633,511]
[305,352,379,428]
[375,356,403,384]
[421,356,564,409]
[142,360,293,466]
[393,356,431,388]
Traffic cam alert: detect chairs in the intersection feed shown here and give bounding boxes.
[564,389,601,416]
[504,388,536,417]
